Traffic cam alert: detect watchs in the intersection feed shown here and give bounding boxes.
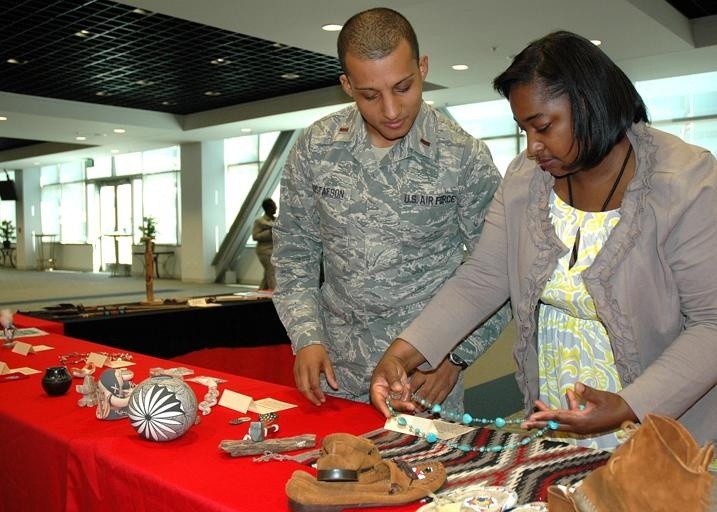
[446,352,469,372]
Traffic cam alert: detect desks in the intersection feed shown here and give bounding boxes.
[2,329,611,510]
[20,291,293,358]
[132,251,174,278]
[0,248,17,269]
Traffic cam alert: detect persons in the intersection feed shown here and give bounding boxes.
[266,6,510,423]
[252,197,278,290]
[368,26,716,450]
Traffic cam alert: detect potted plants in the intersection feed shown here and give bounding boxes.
[0,220,16,248]
[139,216,157,251]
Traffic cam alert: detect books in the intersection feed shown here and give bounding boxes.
[216,295,258,303]
[232,290,272,301]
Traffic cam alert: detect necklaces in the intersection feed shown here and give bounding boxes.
[383,384,561,454]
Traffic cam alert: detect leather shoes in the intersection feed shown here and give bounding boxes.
[286,433,448,511]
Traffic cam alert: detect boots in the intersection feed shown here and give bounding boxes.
[546,413,716,511]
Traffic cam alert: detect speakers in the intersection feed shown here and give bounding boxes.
[0,180,17,201]
